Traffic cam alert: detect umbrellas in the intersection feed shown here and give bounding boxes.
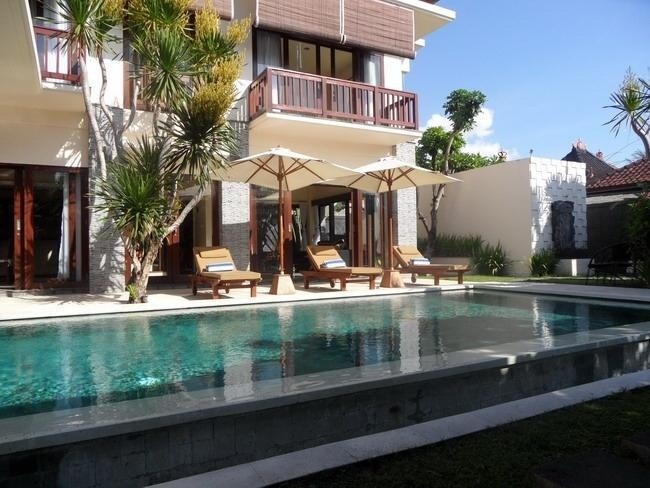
[325,150,465,268]
[201,145,364,276]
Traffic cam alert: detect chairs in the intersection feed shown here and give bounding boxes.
[190,244,470,299]
[584,239,641,287]
